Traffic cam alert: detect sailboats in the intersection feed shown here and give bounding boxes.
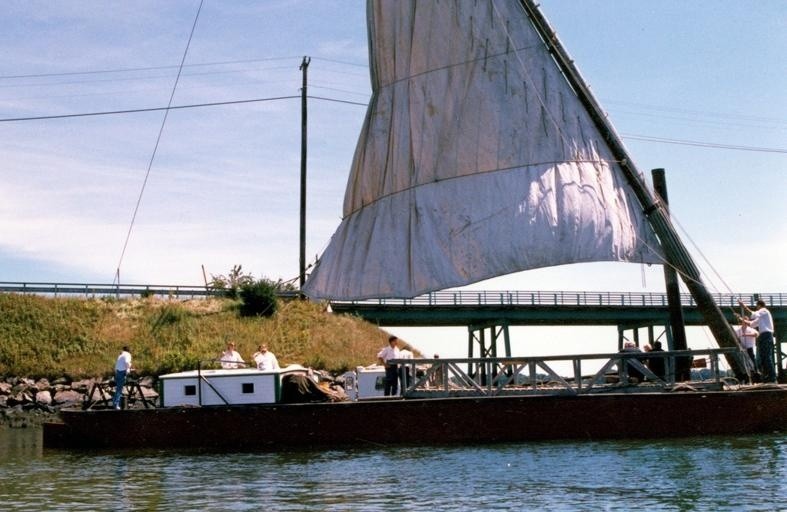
[57,0,787,451]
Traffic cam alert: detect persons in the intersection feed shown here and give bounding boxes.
[399,346,413,395]
[378,335,399,396]
[221,342,247,369]
[253,344,279,370]
[736,301,778,383]
[112,346,132,410]
[612,342,669,380]
[432,354,443,389]
